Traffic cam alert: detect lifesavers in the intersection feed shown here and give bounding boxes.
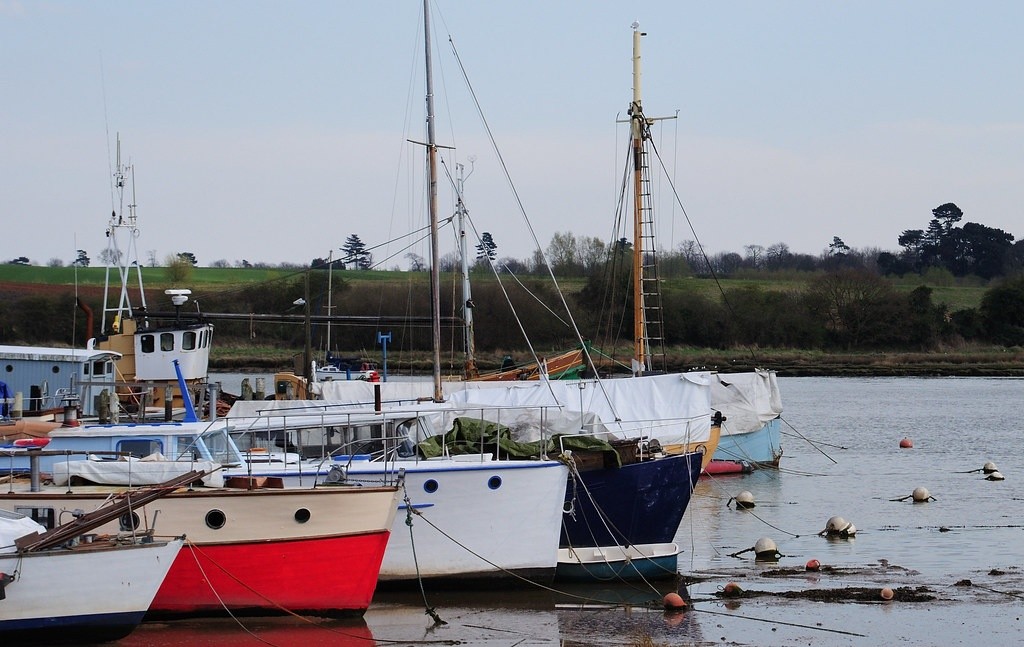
[12,438,49,447]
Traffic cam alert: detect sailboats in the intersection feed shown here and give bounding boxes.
[201,1,785,574]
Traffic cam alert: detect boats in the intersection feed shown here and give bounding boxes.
[0,509,185,646]
[0,438,404,619]
[79,60,214,409]
[0,337,570,591]
